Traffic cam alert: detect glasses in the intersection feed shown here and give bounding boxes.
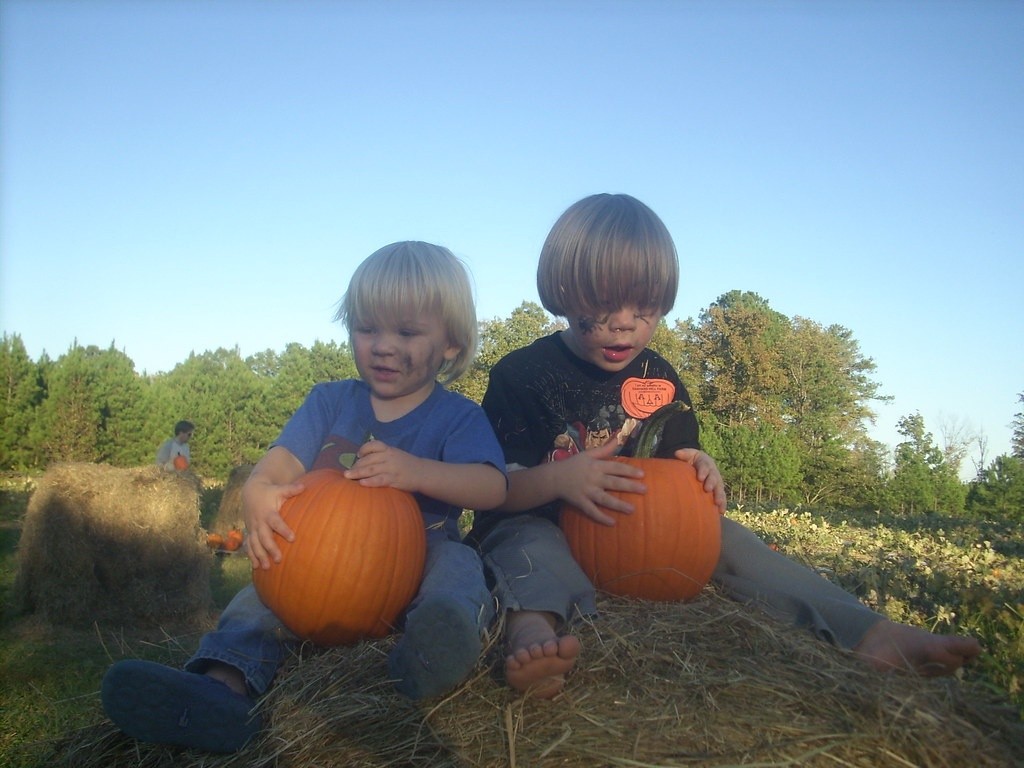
[184,432,191,437]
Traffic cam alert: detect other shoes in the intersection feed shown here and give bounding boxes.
[389,601,480,700]
[101,659,260,754]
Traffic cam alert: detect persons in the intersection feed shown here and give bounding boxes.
[100,241,508,753]
[156,421,194,474]
[460,194,982,699]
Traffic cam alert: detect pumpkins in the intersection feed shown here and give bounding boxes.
[768,543,781,553]
[174,451,189,469]
[206,525,244,550]
[253,432,426,649]
[557,401,720,601]
